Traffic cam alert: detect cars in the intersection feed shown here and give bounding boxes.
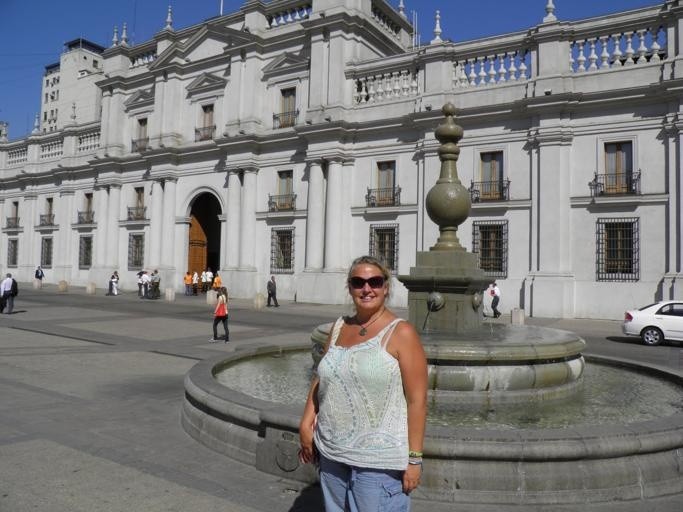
[620,299,683,343]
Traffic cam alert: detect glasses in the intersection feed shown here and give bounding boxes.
[348,276,384,289]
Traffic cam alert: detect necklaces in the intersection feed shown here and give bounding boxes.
[354,307,385,336]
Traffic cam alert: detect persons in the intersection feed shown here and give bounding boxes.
[267,276,280,307]
[209,287,229,344]
[111,267,221,300]
[0,274,14,314]
[34,266,46,289]
[298,256,428,512]
[491,283,501,318]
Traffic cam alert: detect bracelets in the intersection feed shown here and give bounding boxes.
[408,451,423,465]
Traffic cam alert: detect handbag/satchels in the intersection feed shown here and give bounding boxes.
[214,303,226,317]
